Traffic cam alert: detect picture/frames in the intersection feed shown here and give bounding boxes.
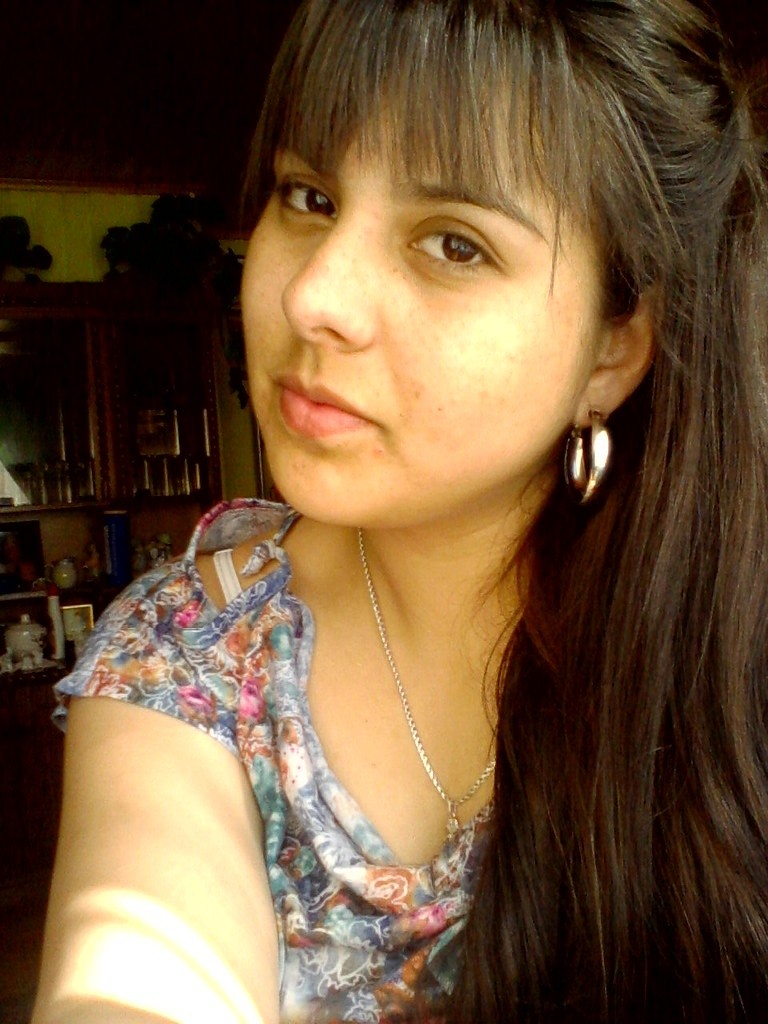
[61,603,95,649]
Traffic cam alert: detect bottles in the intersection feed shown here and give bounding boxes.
[44,585,66,659]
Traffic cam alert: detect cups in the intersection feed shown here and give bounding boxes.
[4,614,47,662]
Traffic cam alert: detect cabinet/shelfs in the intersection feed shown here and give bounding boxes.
[0,281,223,517]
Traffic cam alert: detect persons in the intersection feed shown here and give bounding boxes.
[34,0,768,1024]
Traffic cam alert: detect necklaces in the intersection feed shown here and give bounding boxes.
[355,525,496,833]
